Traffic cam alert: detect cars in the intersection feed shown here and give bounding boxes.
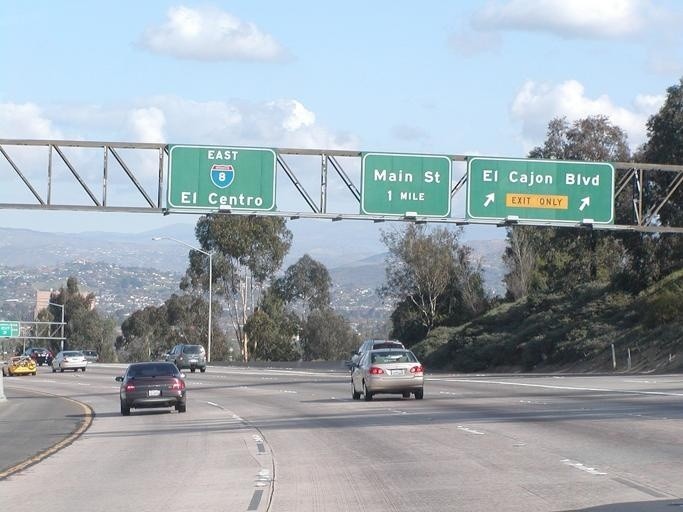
[0,347,99,376]
[349,348,425,399]
[114,361,187,414]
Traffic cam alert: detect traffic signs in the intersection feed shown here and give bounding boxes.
[358,152,452,218]
[165,145,277,212]
[465,156,615,226]
[0,321,20,337]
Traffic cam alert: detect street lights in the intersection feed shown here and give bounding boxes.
[152,235,211,365]
[2,298,64,352]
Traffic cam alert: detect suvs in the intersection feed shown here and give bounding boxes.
[350,338,409,375]
[164,344,205,374]
